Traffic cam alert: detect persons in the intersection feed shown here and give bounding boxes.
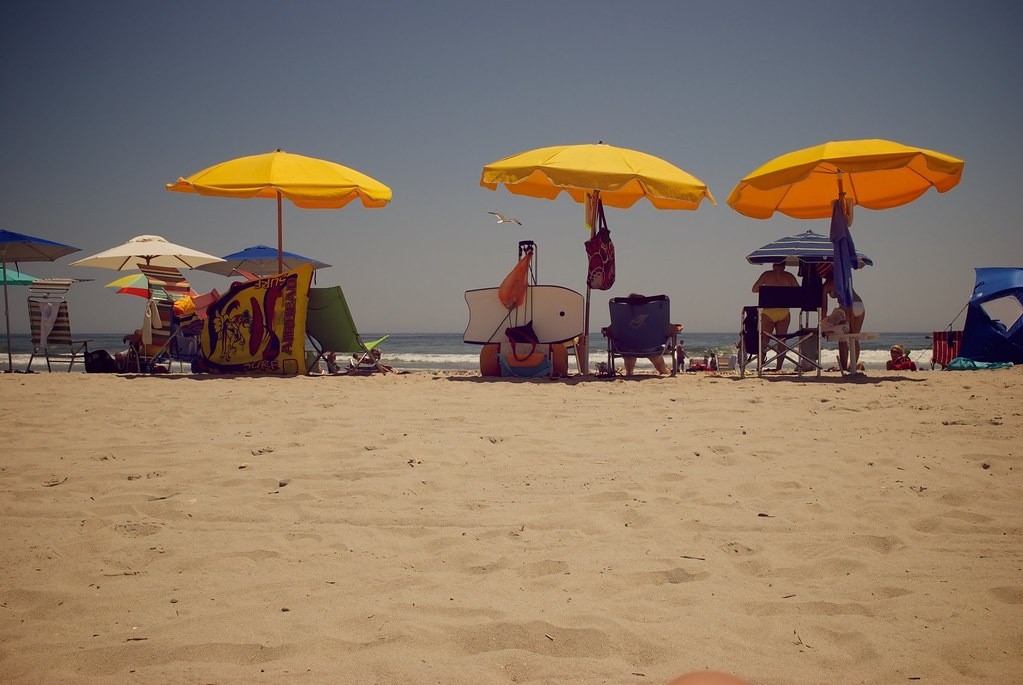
[751,261,800,370]
[616,293,671,377]
[676,339,688,372]
[821,270,865,371]
[703,353,717,370]
[886,345,917,371]
[0,267,41,289]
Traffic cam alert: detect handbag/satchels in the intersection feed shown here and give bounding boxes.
[584,198,615,291]
[498,251,533,309]
[84,350,118,373]
[505,319,539,362]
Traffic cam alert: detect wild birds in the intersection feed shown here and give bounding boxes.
[488,212,522,226]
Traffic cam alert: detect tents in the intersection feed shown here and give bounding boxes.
[912,267,1023,369]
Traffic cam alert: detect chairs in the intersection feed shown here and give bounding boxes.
[930,330,966,370]
[304,286,389,375]
[115,264,199,374]
[758,285,825,379]
[601,293,683,377]
[740,305,757,378]
[26,278,93,373]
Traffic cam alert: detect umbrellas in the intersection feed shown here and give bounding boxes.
[480,139,719,336]
[745,228,873,327]
[726,139,965,374]
[70,232,228,274]
[193,244,333,279]
[1,228,83,372]
[164,146,393,272]
[102,275,197,308]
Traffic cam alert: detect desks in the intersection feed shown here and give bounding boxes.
[827,334,880,377]
